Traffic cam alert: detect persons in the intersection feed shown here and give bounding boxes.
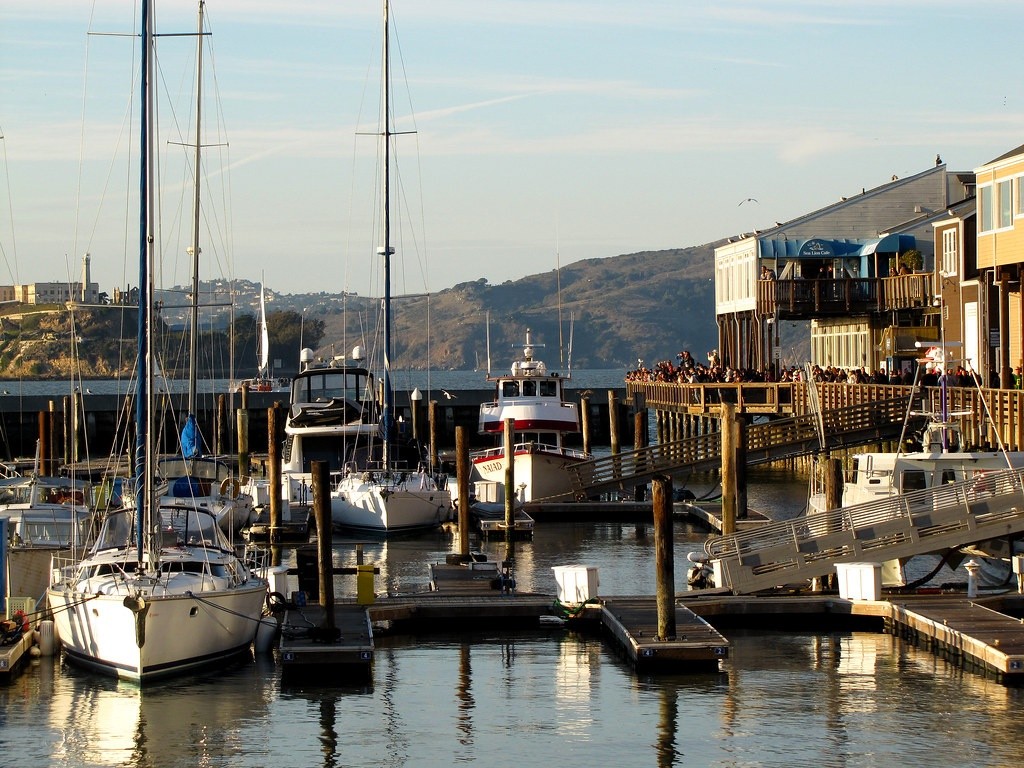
[899,263,912,275]
[15,533,21,543]
[827,265,833,278]
[278,377,291,384]
[780,365,1022,389]
[817,268,825,278]
[889,266,898,277]
[627,349,772,404]
[291,592,298,602]
[188,536,197,544]
[347,465,353,472]
[760,266,776,281]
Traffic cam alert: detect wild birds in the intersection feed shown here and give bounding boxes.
[727,238,734,243]
[775,221,783,227]
[753,229,760,234]
[738,233,748,240]
[738,198,758,206]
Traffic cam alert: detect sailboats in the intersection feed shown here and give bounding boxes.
[0,0,458,677]
[24,0,288,687]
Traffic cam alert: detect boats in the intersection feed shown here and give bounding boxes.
[461,311,603,501]
[805,259,1024,597]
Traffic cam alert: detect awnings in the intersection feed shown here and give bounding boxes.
[757,238,869,258]
[859,235,917,278]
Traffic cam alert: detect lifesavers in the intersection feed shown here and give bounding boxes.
[219,477,239,498]
